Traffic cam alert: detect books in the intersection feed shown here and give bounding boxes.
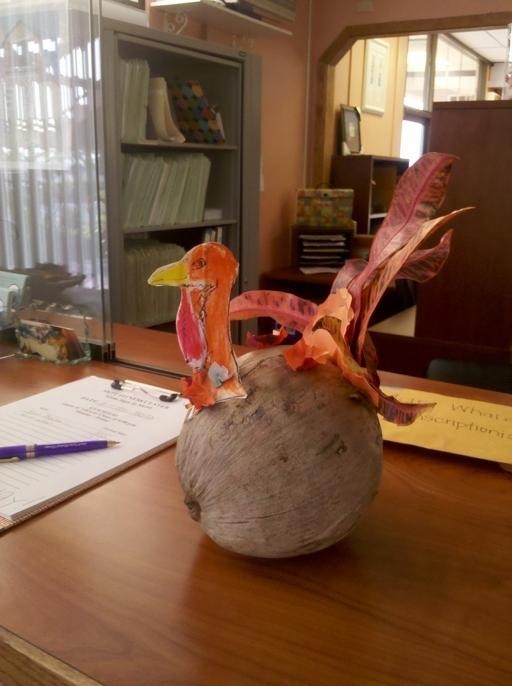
[122,152,211,227]
[201,228,225,245]
[0,374,199,529]
[128,242,185,327]
[299,231,347,267]
[117,56,187,142]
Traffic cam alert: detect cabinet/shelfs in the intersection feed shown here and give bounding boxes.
[331,156,409,234]
[148,0,299,41]
[103,17,258,327]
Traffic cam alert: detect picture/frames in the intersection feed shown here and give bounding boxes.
[361,38,390,118]
[340,105,364,157]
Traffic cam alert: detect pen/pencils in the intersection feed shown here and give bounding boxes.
[0,441,120,463]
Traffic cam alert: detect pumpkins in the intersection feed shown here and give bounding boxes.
[175,345,384,559]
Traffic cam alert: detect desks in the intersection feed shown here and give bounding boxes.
[1,316,511,686]
